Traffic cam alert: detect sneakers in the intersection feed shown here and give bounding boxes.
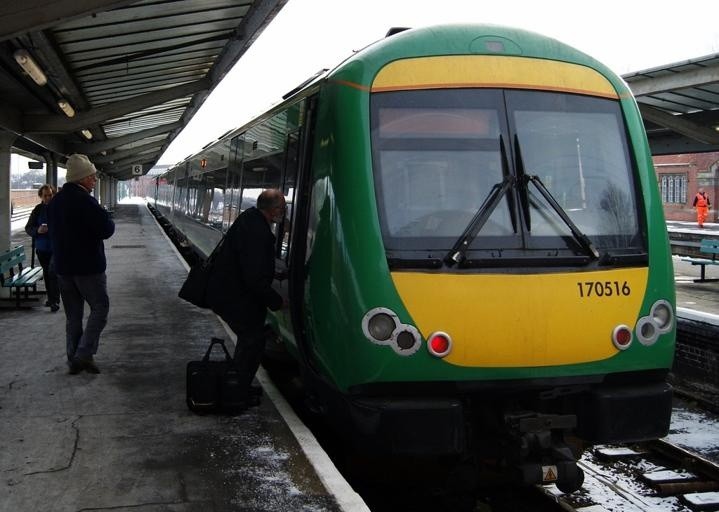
[75,354,99,374]
[68,360,82,373]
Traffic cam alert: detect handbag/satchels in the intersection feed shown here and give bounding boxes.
[178,256,214,309]
[186,337,232,416]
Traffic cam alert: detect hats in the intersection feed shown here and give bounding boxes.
[66,154,97,183]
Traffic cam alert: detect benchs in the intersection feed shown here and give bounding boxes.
[681,240,719,283]
[0,245,44,311]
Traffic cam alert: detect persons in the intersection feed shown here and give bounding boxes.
[693,188,712,228]
[44,154,115,375]
[25,184,61,312]
[204,188,287,408]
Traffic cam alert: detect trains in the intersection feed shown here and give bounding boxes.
[144,24,678,512]
